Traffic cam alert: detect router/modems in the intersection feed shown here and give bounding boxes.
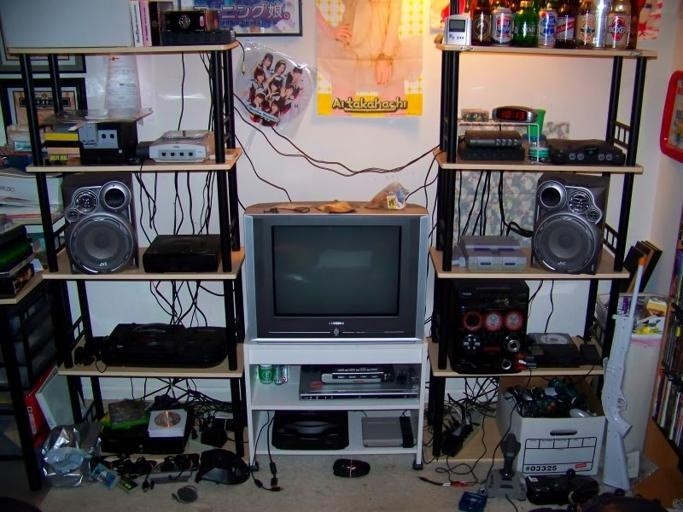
[362,416,413,448]
[149,129,214,163]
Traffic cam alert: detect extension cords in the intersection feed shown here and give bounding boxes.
[188,404,227,452]
[440,415,480,456]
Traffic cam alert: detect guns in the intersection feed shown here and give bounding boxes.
[602,257,647,493]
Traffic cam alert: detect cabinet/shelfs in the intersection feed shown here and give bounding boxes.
[236,326,436,478]
[422,38,655,469]
[7,39,259,482]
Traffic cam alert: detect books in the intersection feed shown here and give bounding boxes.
[129,0,159,49]
[0,125,79,234]
[622,223,683,447]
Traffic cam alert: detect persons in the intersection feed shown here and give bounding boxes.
[316,0,404,116]
[247,53,304,127]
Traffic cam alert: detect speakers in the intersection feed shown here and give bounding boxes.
[60,173,139,273]
[449,280,530,374]
[530,173,612,275]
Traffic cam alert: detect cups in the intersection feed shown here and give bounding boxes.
[526,108,545,135]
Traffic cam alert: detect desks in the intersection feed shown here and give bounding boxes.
[2,236,85,498]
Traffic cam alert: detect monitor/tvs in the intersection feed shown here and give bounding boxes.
[243,203,430,340]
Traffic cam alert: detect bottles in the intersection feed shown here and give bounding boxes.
[530,136,549,164]
[472,0,632,52]
[256,365,288,385]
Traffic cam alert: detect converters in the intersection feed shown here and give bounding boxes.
[442,434,463,457]
[200,426,228,448]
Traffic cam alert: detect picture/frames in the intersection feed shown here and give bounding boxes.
[657,69,682,165]
[1,46,86,74]
[175,0,305,38]
[0,76,88,143]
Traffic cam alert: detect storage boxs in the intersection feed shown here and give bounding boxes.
[596,289,669,461]
[488,388,609,479]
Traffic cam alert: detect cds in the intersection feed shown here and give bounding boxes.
[541,333,569,344]
[154,411,181,428]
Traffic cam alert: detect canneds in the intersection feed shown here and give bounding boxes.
[537,8,556,49]
[607,11,628,49]
[491,8,514,47]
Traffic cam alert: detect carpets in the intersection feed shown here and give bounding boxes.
[38,397,607,511]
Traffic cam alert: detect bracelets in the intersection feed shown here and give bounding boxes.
[379,53,392,62]
